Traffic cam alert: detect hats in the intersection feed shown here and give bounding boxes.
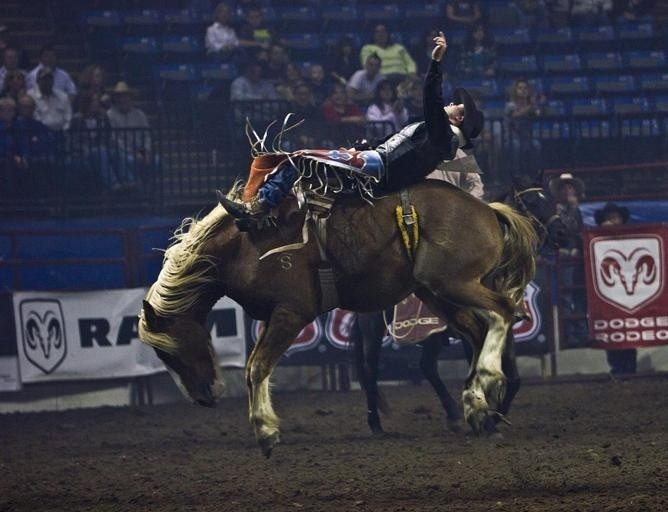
[593,202,630,226]
[453,87,485,150]
[548,173,585,200]
[104,81,140,96]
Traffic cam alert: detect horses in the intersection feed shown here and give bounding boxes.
[136,177,541,461]
[345,166,572,439]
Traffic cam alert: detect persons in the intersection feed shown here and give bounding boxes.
[541,174,638,374]
[0,46,160,196]
[446,1,500,80]
[204,2,425,147]
[511,1,639,22]
[215,32,481,231]
[505,78,548,120]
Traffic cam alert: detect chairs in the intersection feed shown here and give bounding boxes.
[0,1,667,212]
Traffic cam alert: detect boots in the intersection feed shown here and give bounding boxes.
[215,188,272,233]
